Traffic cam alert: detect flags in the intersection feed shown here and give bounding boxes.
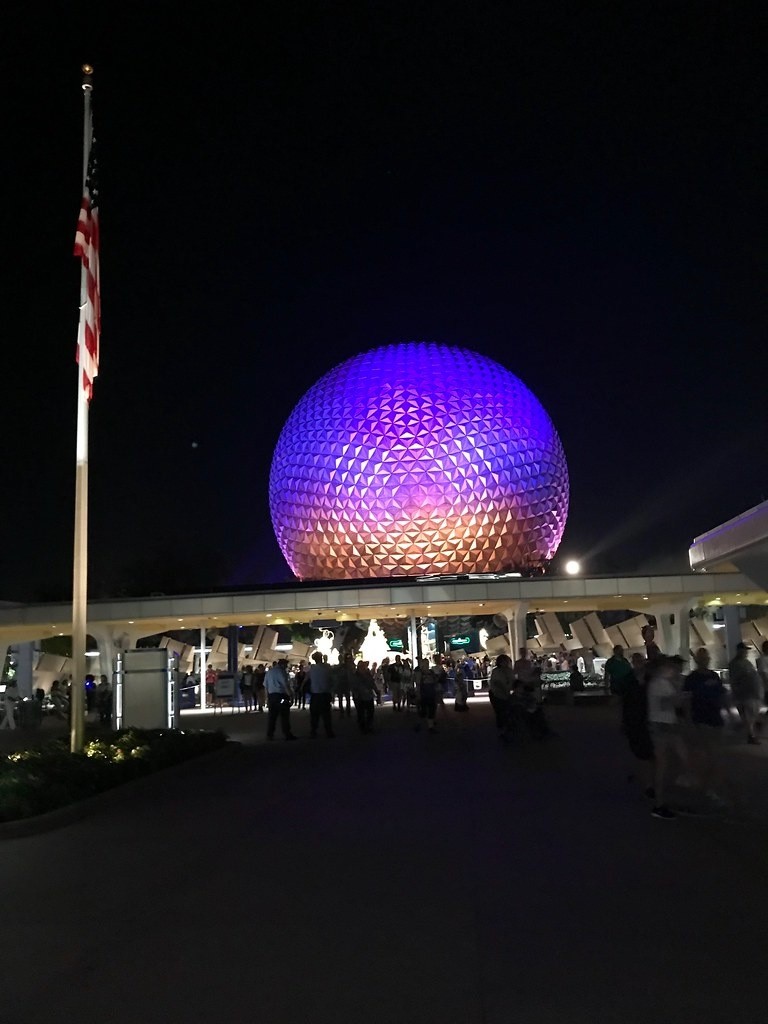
[72,112,104,402]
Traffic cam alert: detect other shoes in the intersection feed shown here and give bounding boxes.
[669,805,696,816]
[651,804,677,820]
[747,737,761,745]
[428,728,439,734]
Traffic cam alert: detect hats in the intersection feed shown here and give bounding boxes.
[737,642,752,649]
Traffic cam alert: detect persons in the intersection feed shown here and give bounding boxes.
[349,660,382,737]
[0,679,23,731]
[174,653,491,718]
[263,658,295,744]
[488,642,768,827]
[300,652,336,740]
[51,673,114,731]
[415,658,441,733]
